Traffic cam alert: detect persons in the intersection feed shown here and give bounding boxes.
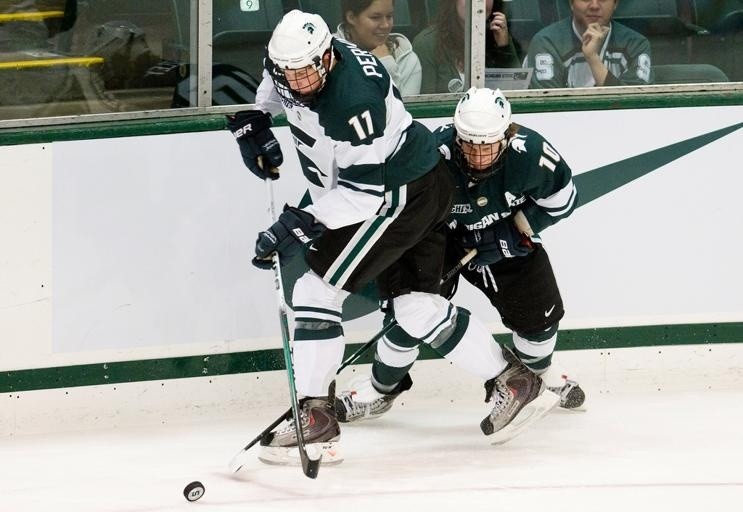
[82,17,260,104]
[226,7,562,468]
[412,0,521,95]
[332,1,423,96]
[524,0,655,88]
[334,85,586,424]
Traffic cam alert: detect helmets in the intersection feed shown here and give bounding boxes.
[269,10,335,109]
[452,86,512,185]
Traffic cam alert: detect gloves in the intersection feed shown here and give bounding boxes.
[225,111,283,181]
[462,217,534,267]
[251,203,328,270]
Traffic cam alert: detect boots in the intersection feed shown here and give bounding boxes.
[480,342,545,434]
[259,382,340,447]
[541,371,585,408]
[335,372,413,423]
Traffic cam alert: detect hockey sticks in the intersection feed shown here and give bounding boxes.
[263,156,323,477]
[231,248,477,473]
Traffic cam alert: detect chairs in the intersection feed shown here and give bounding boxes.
[508,16,543,56]
[391,25,420,42]
[618,14,704,65]
[722,12,742,79]
[213,28,276,84]
